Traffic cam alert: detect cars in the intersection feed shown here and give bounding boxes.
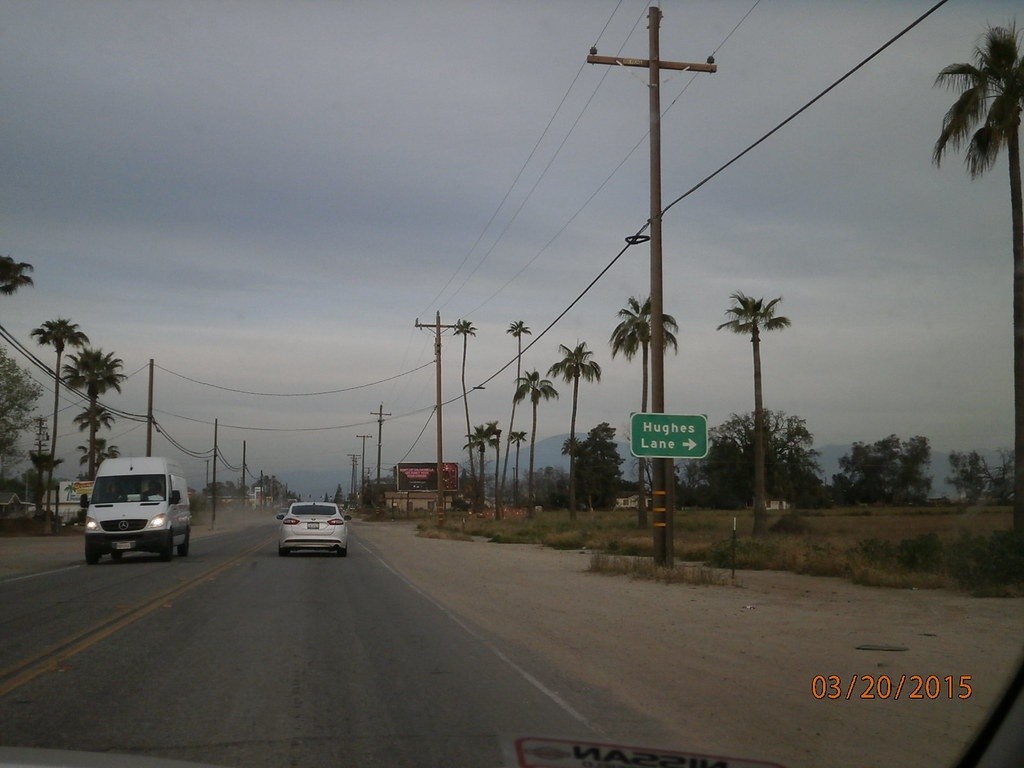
[276,502,352,557]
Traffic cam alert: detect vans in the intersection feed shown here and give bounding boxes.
[80,456,191,565]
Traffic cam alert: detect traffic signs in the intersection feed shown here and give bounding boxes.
[629,411,708,458]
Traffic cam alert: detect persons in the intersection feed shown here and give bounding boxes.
[144,482,164,499]
